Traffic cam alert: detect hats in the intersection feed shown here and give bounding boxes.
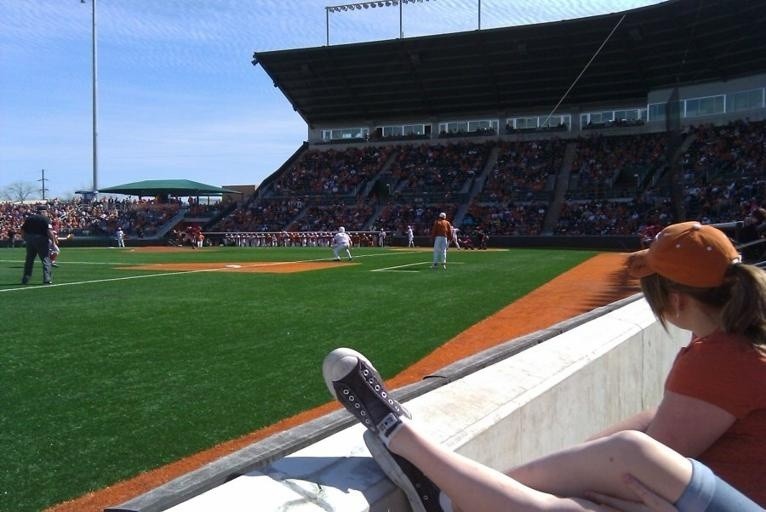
[625,221,742,295]
[440,212,446,219]
[37,205,47,212]
[339,226,346,233]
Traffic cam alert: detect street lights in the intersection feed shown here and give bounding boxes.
[80,0,99,197]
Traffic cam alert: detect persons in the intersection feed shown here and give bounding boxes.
[20,206,59,283]
[235,115,766,248]
[317,347,761,512]
[0,180,236,252]
[574,218,765,502]
[331,226,353,261]
[432,211,453,271]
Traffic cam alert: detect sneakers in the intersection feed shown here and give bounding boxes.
[323,345,409,439]
[333,258,354,262]
[362,428,448,510]
[430,264,447,270]
[44,281,52,284]
